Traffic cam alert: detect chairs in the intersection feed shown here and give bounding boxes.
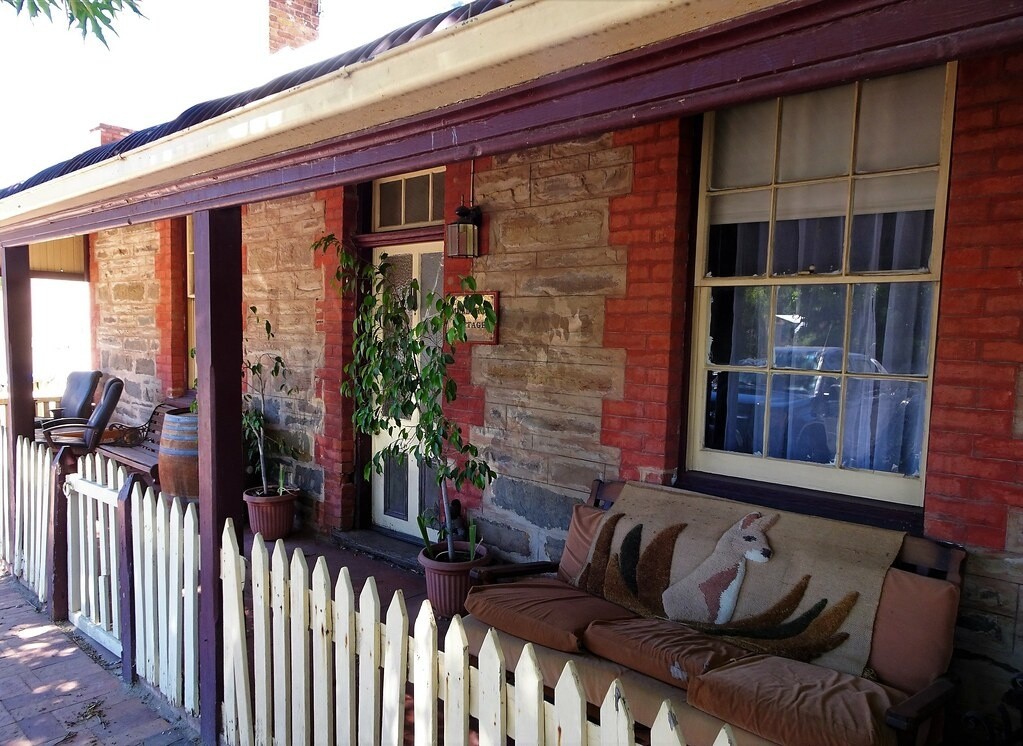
[35,367,189,488]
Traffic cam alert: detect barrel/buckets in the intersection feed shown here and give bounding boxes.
[158,406,199,533]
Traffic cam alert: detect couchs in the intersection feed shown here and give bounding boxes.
[454,477,970,742]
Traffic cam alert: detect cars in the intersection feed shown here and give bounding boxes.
[707,345,913,467]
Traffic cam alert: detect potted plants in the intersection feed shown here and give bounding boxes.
[193,305,305,541]
[311,236,500,620]
